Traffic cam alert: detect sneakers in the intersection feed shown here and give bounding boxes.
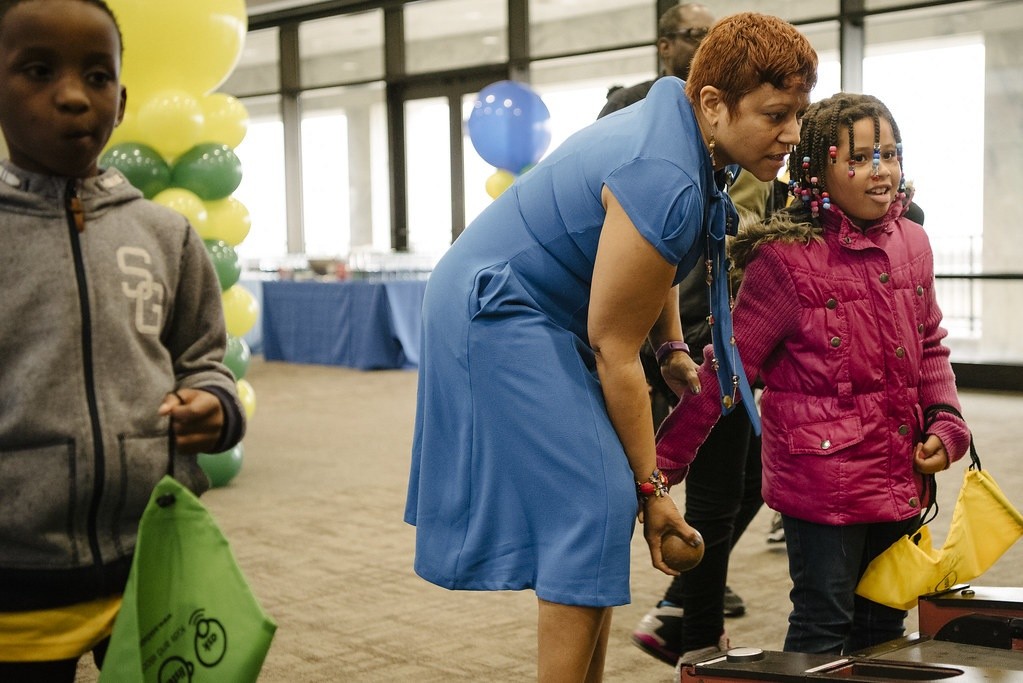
[724,586,745,617]
[767,514,785,544]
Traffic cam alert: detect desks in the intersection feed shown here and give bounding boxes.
[260,278,426,373]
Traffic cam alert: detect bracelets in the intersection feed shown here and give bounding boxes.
[656,341,691,364]
[636,469,668,503]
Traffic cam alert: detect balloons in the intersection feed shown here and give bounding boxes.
[98,0,256,487]
[486,171,514,198]
[469,80,550,173]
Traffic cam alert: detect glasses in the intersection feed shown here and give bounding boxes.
[663,26,708,42]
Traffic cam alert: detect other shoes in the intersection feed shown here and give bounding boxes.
[632,601,730,668]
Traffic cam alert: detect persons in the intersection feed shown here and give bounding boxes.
[403,14,817,682]
[0,0,246,683]
[601,0,972,674]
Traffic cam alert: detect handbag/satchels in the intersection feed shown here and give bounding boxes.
[854,403,1023,610]
[99,393,279,683]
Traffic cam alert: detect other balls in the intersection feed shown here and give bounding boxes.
[662,526,705,572]
